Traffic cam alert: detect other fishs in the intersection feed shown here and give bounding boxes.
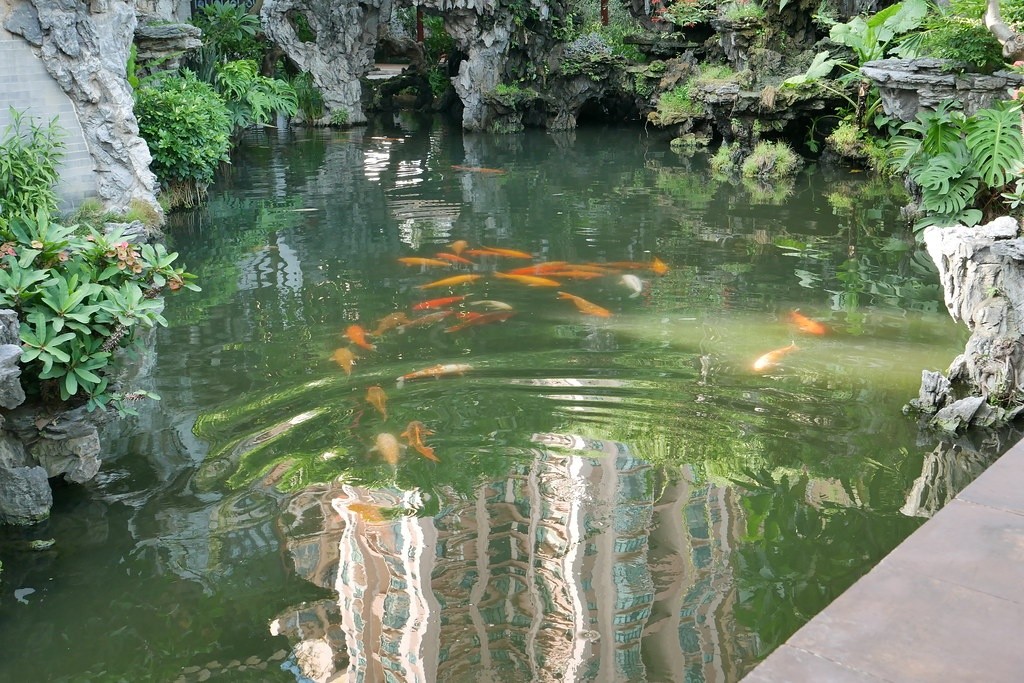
[751,306,829,371]
[238,125,667,479]
